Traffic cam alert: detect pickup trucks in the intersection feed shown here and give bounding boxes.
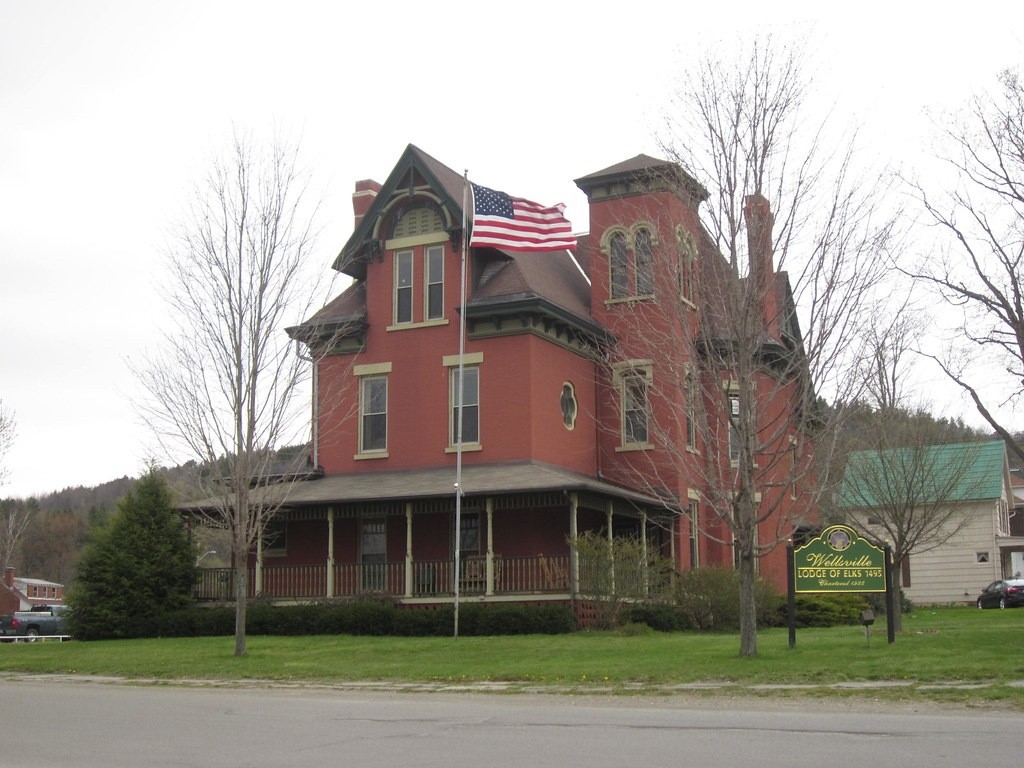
[0,605,72,644]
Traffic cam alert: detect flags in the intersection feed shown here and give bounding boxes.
[469,183,577,252]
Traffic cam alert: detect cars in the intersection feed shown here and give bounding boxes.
[977,579,1024,610]
